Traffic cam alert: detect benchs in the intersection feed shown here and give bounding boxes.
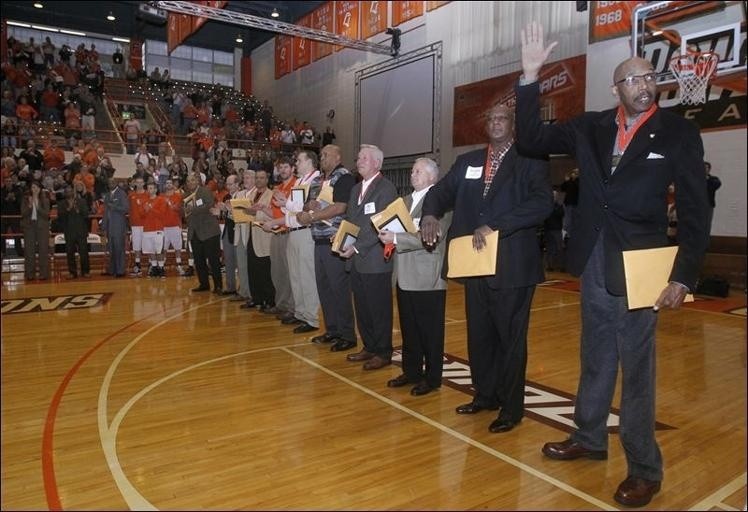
[0,65,297,156]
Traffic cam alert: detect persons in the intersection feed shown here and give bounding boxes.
[2,36,317,283]
[331,142,394,370]
[371,157,452,398]
[540,161,721,270]
[419,102,552,434]
[181,144,358,350]
[514,18,710,507]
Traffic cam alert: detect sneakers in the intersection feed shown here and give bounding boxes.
[128,266,195,277]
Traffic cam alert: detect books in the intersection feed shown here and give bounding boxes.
[332,218,358,257]
[366,198,415,238]
[622,245,695,309]
[446,229,498,278]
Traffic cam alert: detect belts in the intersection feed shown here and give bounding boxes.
[287,225,312,232]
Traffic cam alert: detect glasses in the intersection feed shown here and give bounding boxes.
[616,73,658,86]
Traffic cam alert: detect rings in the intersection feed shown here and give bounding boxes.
[382,239,386,242]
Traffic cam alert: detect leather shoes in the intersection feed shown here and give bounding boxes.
[363,355,392,370]
[67,274,76,280]
[293,323,319,333]
[82,273,90,277]
[312,333,340,343]
[489,415,515,432]
[542,439,607,460]
[614,475,661,507]
[192,287,209,291]
[456,403,478,414]
[213,288,250,302]
[241,299,305,325]
[331,340,357,352]
[347,348,375,362]
[387,373,421,388]
[411,382,433,396]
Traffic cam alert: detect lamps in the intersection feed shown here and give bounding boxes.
[105,10,118,23]
[32,0,47,11]
[381,25,405,56]
[233,28,243,43]
[269,3,281,19]
